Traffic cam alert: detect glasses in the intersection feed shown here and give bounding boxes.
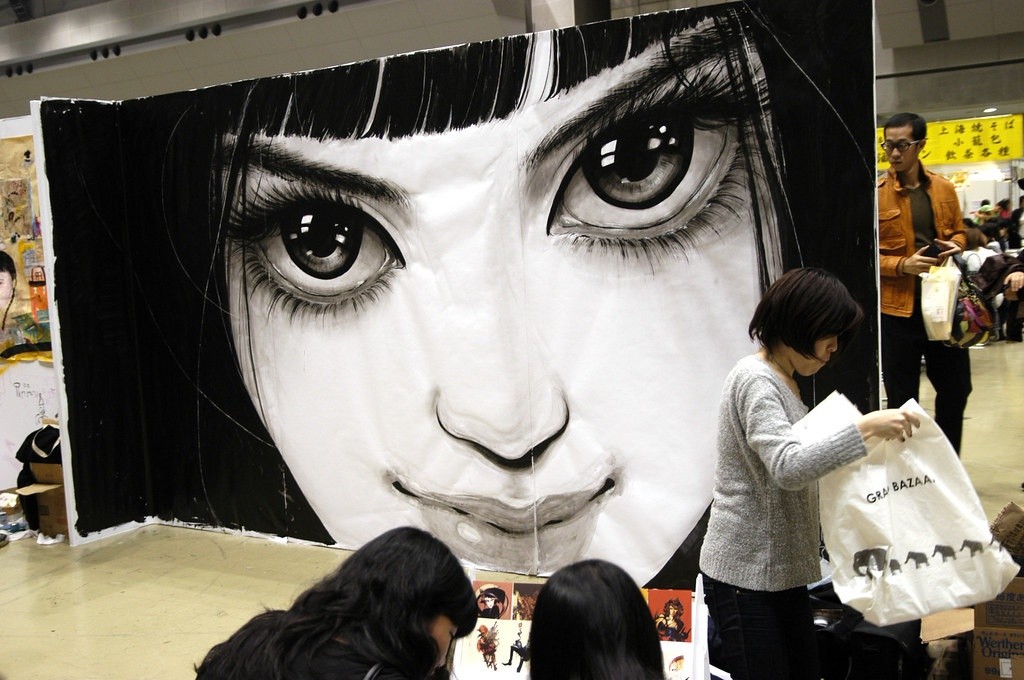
[881,140,922,152]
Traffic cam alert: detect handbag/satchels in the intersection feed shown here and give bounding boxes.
[817,397,1019,628]
[15,426,62,465]
[918,255,962,340]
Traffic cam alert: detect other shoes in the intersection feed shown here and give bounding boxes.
[0,533,8,547]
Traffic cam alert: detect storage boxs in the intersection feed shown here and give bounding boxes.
[970,568,1024,680]
[18,465,70,540]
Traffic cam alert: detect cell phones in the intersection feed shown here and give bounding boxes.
[921,243,942,258]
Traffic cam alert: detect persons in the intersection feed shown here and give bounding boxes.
[194,524,478,680]
[963,178,1024,344]
[877,112,972,458]
[527,558,665,679]
[699,269,920,680]
